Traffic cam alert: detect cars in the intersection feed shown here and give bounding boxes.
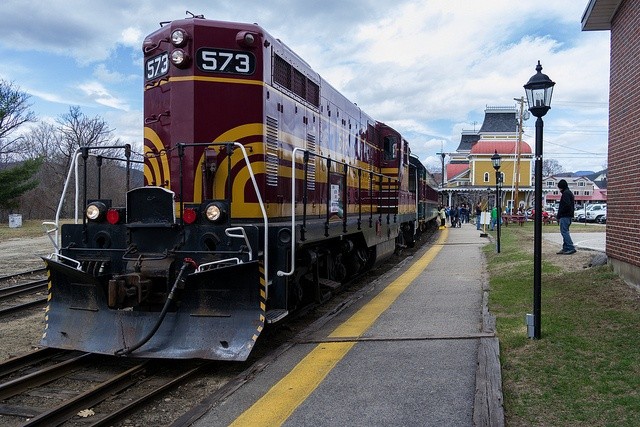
[585,207,607,224]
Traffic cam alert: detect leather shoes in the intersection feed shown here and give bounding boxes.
[556,250,562,253]
[563,249,575,253]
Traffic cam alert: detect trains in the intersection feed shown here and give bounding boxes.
[40,11,440,364]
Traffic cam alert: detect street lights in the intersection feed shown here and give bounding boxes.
[522,60,555,341]
[492,149,503,253]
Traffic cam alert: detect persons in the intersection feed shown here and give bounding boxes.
[556,179,576,255]
[439,203,550,231]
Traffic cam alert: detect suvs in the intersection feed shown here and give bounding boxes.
[573,204,606,223]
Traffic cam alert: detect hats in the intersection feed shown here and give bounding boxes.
[557,179,567,188]
